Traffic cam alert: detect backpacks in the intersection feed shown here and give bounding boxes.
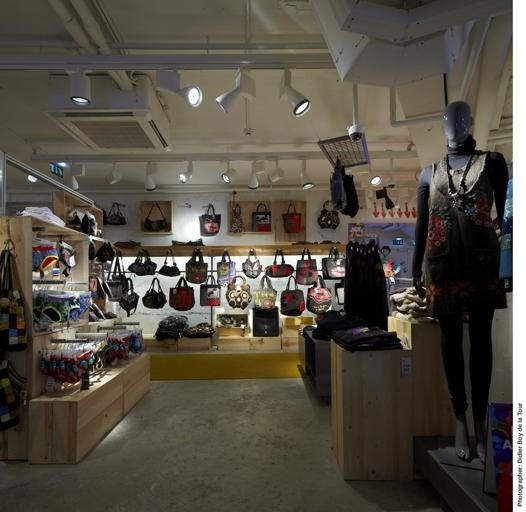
[252,308,278,338]
[154,315,188,339]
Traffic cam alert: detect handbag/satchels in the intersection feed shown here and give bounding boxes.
[281,204,301,233]
[107,249,208,317]
[202,204,221,234]
[252,204,271,232]
[200,247,346,316]
[318,201,340,229]
[230,205,243,232]
[106,202,126,225]
[0,247,27,351]
[144,202,167,231]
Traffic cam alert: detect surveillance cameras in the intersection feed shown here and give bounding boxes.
[349,126,362,142]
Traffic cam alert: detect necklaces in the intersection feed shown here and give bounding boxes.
[445,153,474,209]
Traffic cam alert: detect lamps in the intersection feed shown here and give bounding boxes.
[1,58,336,119]
[26,151,385,191]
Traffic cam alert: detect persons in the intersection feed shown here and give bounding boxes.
[409,100,509,463]
[380,245,401,315]
